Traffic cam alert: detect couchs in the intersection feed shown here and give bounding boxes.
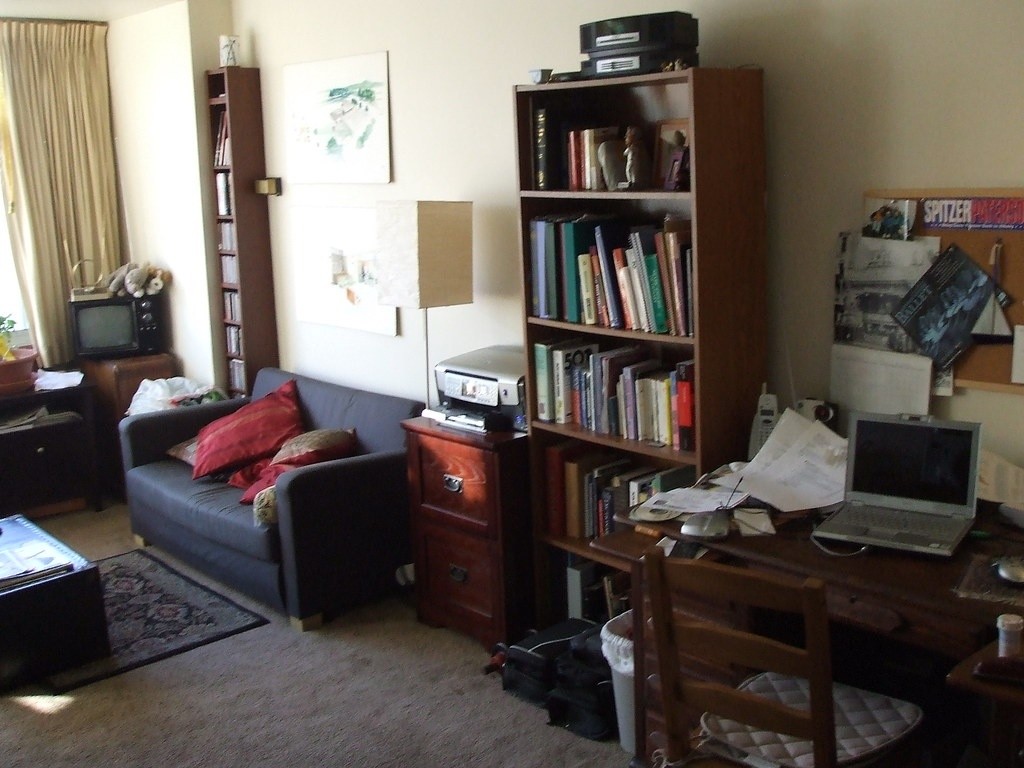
[119,369,426,631]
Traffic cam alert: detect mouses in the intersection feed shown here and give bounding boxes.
[992,555,1024,586]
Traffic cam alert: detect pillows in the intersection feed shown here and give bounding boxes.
[165,380,360,521]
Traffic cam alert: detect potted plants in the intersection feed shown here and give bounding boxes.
[0,314,38,385]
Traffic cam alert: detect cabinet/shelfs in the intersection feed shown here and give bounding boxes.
[406,418,528,655]
[512,67,766,635]
[203,66,280,399]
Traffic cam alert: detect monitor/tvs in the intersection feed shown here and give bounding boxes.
[67,295,166,361]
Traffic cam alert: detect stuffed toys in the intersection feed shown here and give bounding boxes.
[107,260,171,298]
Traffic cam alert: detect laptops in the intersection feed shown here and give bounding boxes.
[812,413,982,555]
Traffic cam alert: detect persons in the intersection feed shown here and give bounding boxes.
[870,205,904,239]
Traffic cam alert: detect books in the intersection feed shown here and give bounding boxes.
[0,542,73,591]
[535,109,689,191]
[556,548,630,621]
[565,454,694,539]
[529,218,694,338]
[534,343,695,450]
[214,111,247,394]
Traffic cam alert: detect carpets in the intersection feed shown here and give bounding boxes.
[38,550,273,697]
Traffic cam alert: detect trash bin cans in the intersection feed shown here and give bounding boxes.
[601,608,636,754]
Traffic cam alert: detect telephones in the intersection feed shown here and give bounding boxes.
[748,381,779,461]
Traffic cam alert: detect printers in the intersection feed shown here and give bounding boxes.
[422,345,528,433]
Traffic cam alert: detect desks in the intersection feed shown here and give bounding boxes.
[0,515,100,694]
[606,504,1024,768]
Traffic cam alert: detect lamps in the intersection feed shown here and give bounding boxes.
[377,200,474,412]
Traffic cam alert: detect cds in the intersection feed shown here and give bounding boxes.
[635,506,683,521]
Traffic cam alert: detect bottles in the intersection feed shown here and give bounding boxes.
[996,613,1024,658]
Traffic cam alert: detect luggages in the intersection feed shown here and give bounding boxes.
[493,618,618,742]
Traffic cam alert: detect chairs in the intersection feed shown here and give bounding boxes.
[645,550,923,768]
